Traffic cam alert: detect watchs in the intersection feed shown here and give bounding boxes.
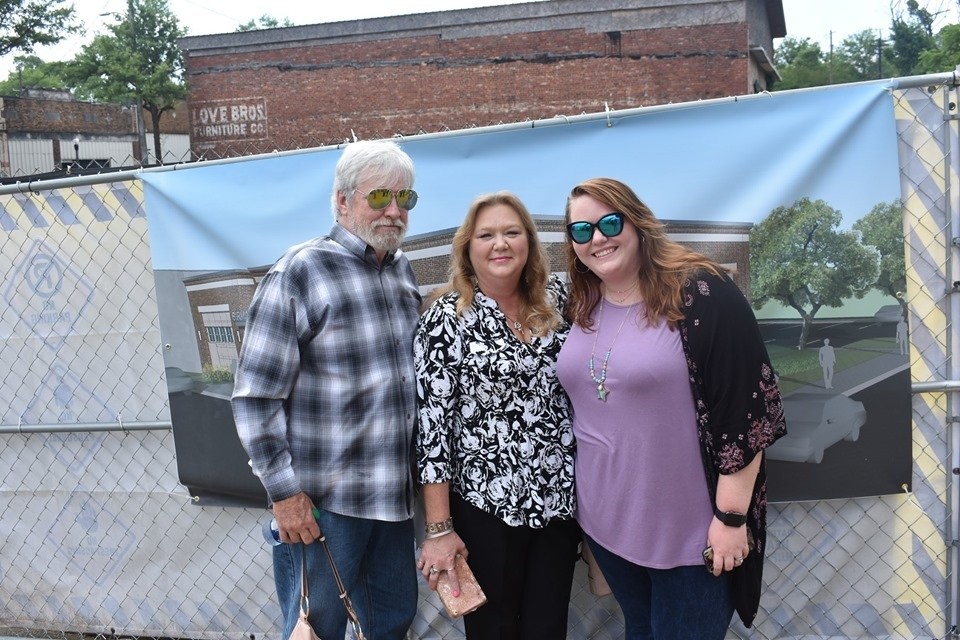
[713,507,748,528]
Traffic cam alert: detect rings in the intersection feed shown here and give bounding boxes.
[447,566,456,573]
[429,567,440,574]
[417,561,424,566]
[737,556,744,564]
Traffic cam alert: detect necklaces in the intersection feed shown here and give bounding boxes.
[607,284,638,304]
[589,282,641,403]
[606,278,638,293]
[500,309,529,337]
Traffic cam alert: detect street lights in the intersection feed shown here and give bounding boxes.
[72,137,80,169]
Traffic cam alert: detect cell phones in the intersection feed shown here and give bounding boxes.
[703,526,755,573]
[435,552,487,620]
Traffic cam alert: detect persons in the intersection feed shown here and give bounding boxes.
[231,140,422,640]
[414,191,578,639]
[555,178,788,640]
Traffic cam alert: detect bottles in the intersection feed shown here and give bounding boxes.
[262,507,321,546]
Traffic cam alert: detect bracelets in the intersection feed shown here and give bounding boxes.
[425,529,454,539]
[424,517,455,534]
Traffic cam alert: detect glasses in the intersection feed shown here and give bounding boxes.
[566,212,626,245]
[355,188,419,211]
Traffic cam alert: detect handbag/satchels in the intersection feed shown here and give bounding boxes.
[288,530,367,640]
